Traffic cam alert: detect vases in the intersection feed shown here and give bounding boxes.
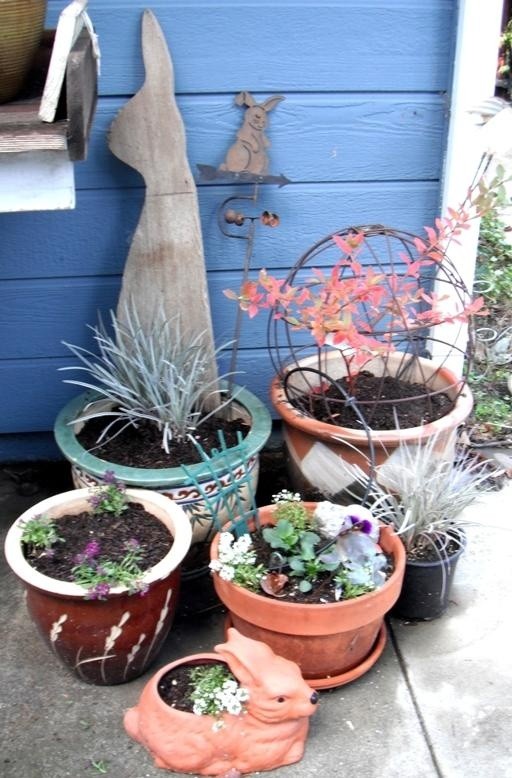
[5,486,192,685]
[210,502,405,677]
[123,628,320,778]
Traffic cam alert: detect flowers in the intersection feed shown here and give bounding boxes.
[83,470,124,507]
[207,488,383,589]
[190,678,250,717]
[66,537,151,600]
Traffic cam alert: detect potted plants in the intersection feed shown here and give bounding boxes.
[223,151,508,501]
[342,406,504,619]
[55,292,273,546]
